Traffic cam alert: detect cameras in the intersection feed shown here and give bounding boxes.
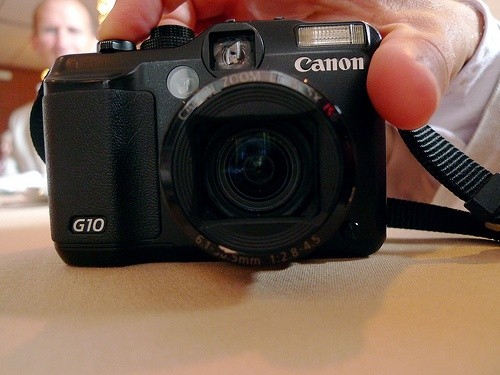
[42,16,387,267]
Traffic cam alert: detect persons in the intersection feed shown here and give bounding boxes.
[0,0,99,200]
[96,0,500,212]
